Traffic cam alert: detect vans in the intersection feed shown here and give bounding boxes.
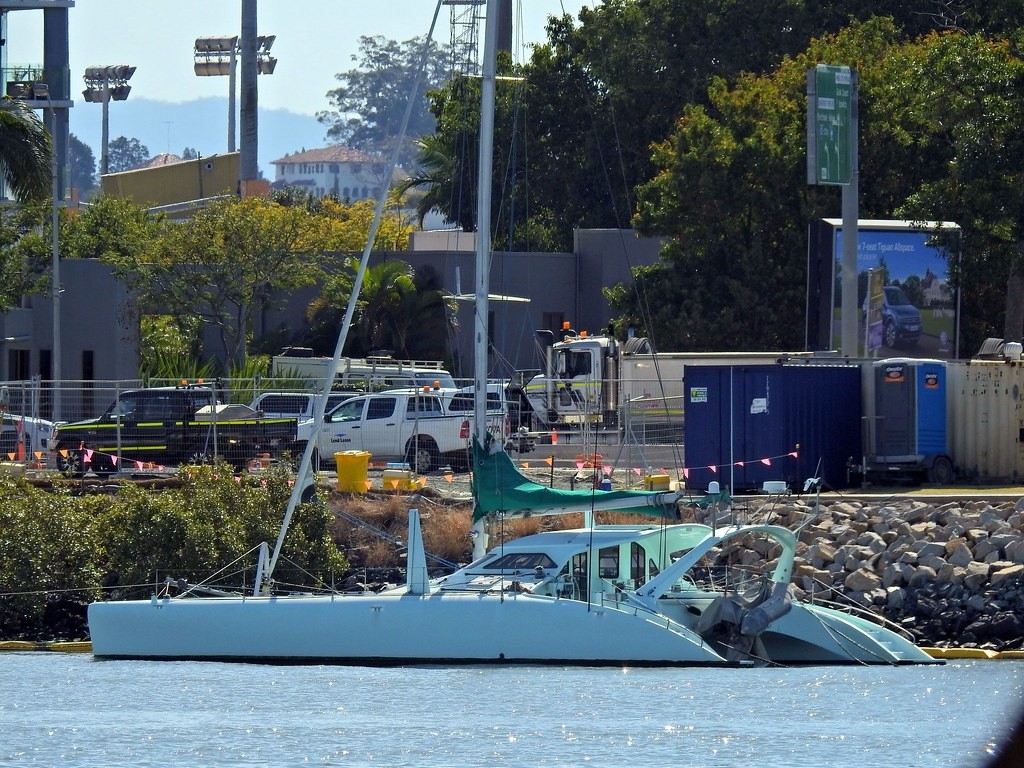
[863,286,922,349]
[247,392,360,447]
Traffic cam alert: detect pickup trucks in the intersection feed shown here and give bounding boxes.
[296,382,510,476]
[49,381,299,480]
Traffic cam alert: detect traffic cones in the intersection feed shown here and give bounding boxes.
[551,427,558,446]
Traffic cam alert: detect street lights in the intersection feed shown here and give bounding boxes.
[192,34,278,153]
[81,63,137,174]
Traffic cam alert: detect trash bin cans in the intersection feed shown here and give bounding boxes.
[332,449,372,494]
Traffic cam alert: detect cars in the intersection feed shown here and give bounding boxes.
[0,411,69,465]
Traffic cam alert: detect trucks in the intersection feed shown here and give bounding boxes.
[521,320,843,444]
[271,346,456,391]
